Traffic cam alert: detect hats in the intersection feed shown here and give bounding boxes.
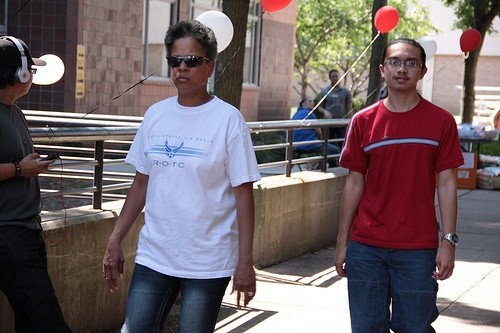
[0,38,46,66]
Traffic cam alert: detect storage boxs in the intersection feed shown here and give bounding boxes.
[436,152,479,188]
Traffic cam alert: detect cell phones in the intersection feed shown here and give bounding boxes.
[39,153,60,162]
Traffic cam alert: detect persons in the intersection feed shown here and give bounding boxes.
[317,69,354,150]
[335,39,465,333]
[292,98,341,169]
[0,36,71,333]
[103,19,261,333]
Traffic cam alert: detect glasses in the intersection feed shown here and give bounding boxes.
[166,56,211,67]
[29,68,37,74]
[383,59,425,68]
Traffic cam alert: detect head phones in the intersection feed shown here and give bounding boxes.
[0,36,30,83]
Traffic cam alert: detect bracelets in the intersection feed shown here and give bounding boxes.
[14,161,21,177]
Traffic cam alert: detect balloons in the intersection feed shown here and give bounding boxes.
[417,36,437,60]
[374,5,399,33]
[460,29,481,52]
[195,10,234,53]
[31,54,65,85]
[259,0,292,11]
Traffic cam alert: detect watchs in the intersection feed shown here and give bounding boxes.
[441,233,459,246]
